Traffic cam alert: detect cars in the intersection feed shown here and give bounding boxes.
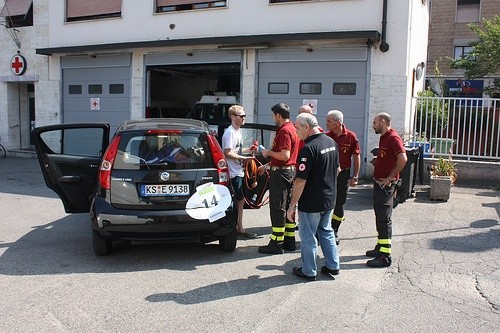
[31,118,271,256]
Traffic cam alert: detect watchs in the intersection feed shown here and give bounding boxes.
[289,204,295,210]
[353,176,358,182]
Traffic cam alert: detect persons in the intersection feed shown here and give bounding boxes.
[223,103,361,254]
[366,112,408,267]
[287,113,341,279]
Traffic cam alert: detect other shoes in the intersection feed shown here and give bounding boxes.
[367,258,391,267]
[237,230,257,237]
[258,239,296,254]
[366,249,380,257]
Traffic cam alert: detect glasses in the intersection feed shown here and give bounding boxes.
[234,115,246,118]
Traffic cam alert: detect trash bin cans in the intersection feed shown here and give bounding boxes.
[410,141,430,157]
[430,137,455,159]
[374,146,419,199]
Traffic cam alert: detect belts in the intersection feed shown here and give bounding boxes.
[341,168,349,171]
[272,166,294,171]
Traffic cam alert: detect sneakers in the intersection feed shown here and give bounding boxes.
[293,266,317,280]
[320,265,339,275]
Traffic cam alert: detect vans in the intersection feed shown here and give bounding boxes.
[186,94,239,144]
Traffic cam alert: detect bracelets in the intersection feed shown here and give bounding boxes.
[387,177,390,182]
